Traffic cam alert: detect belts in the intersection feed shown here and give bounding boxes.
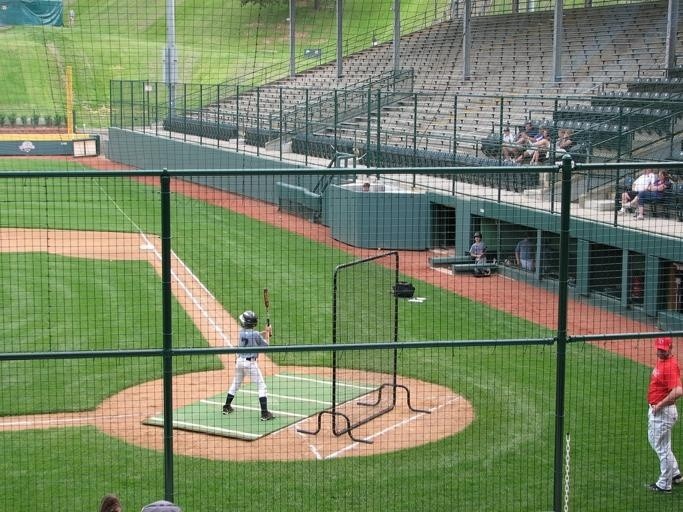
[246,357,257,361]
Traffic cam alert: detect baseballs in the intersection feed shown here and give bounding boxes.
[146,85,152,92]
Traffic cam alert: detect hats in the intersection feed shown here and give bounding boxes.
[655,335,672,351]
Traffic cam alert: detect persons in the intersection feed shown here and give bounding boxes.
[502,120,573,169]
[222,311,273,421]
[470,232,490,276]
[646,337,683,494]
[515,237,534,271]
[362,183,370,191]
[618,168,671,219]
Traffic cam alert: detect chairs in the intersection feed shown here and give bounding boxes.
[478,66,682,221]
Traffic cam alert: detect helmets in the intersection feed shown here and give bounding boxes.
[473,232,482,240]
[239,311,258,329]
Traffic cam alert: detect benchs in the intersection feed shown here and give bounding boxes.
[176,1,683,157]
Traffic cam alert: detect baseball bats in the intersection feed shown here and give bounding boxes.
[264,289,272,337]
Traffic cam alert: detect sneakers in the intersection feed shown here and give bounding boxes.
[616,202,644,220]
[222,405,233,414]
[260,411,277,421]
[643,474,683,494]
